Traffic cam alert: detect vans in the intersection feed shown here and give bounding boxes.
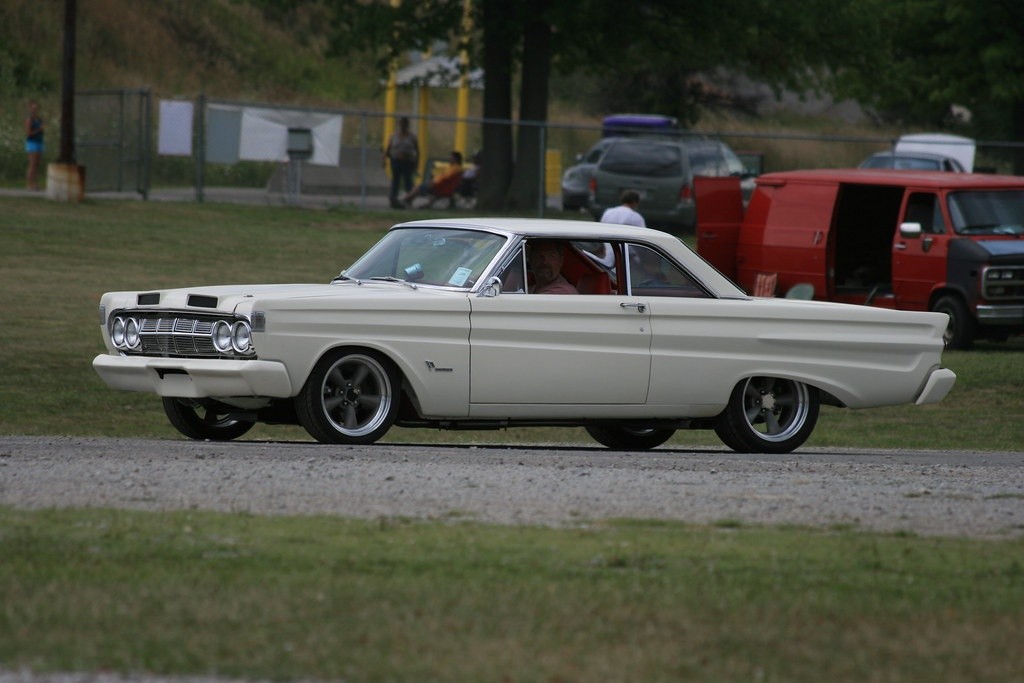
[690,166,1024,347]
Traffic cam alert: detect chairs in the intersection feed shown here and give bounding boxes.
[422,179,463,211]
[577,269,611,296]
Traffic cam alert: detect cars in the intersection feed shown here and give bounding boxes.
[857,134,975,173]
[560,130,760,233]
[94,215,957,456]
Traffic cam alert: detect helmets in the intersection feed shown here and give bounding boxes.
[521,240,566,290]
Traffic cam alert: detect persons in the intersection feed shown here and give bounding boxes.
[23,102,45,191]
[522,239,579,294]
[601,191,646,262]
[385,117,485,209]
